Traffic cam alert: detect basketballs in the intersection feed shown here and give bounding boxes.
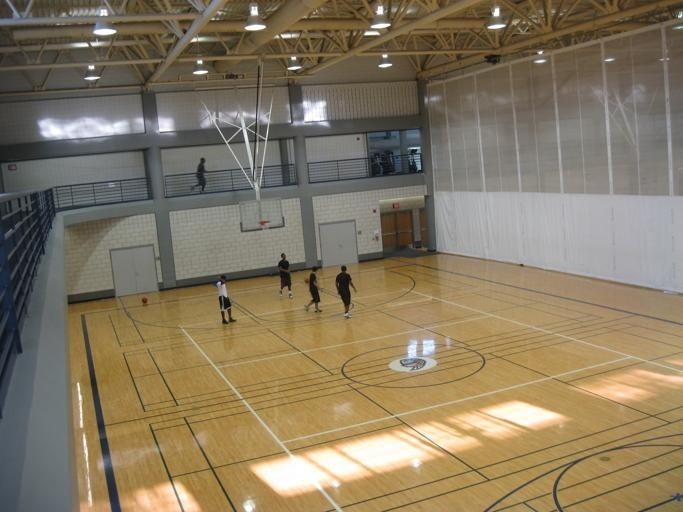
[304,278,309,282]
[142,297,148,303]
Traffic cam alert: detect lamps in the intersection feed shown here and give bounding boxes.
[84,65,103,81]
[371,1,391,29]
[193,38,209,75]
[484,1,507,31]
[243,1,267,32]
[285,30,303,71]
[93,8,117,36]
[378,44,392,68]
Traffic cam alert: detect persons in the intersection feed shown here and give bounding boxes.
[192,158,208,192]
[304,266,323,313]
[278,252,293,299]
[373,153,395,175]
[217,275,236,324]
[336,266,357,318]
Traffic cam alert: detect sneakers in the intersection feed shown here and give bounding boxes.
[222,319,227,324]
[229,318,235,322]
[288,292,292,298]
[314,308,322,312]
[303,304,308,311]
[280,291,282,297]
[343,313,351,318]
